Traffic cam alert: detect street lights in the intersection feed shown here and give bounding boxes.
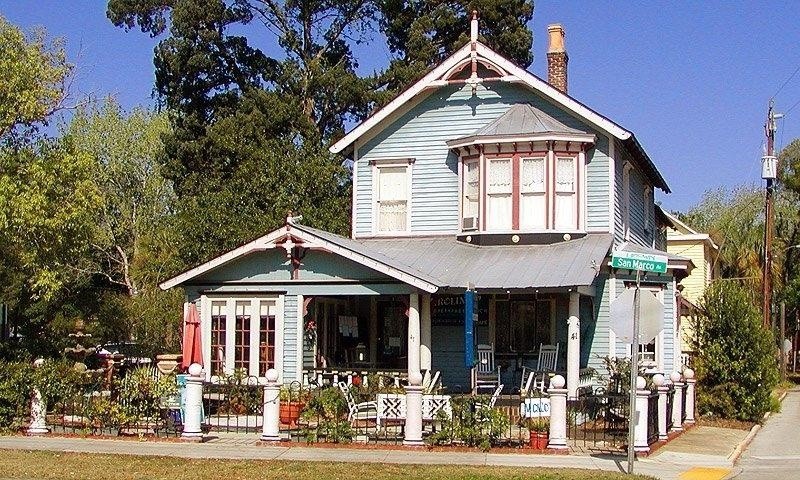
[766,244,800,327]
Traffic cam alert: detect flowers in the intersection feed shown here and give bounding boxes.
[305,315,317,348]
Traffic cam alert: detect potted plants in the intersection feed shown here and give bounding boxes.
[521,418,548,449]
[280,387,310,422]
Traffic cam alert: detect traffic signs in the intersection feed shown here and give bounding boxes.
[613,251,666,272]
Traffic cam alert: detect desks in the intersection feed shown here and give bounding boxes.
[376,393,454,435]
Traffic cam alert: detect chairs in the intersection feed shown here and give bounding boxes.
[338,380,377,428]
[470,343,502,397]
[467,384,505,436]
[519,343,561,395]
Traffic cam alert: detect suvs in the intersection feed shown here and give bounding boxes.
[84,342,183,379]
